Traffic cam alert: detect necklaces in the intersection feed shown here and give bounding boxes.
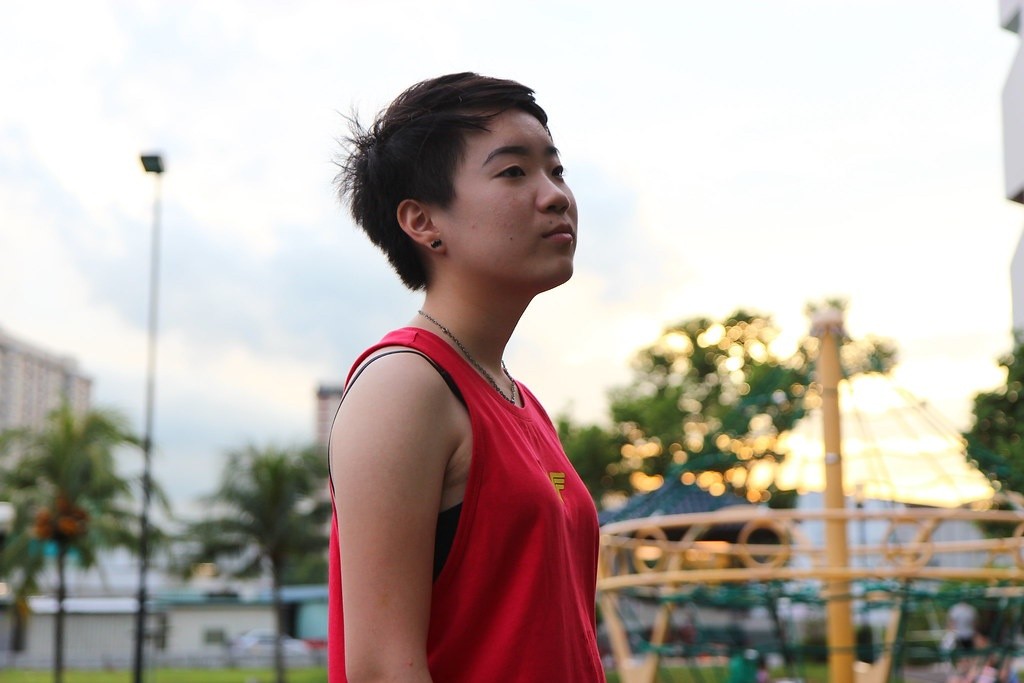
[417,310,516,404]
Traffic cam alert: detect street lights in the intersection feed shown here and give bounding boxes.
[129,150,168,683]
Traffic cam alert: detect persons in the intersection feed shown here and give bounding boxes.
[943,598,1024,683]
[327,71,605,683]
